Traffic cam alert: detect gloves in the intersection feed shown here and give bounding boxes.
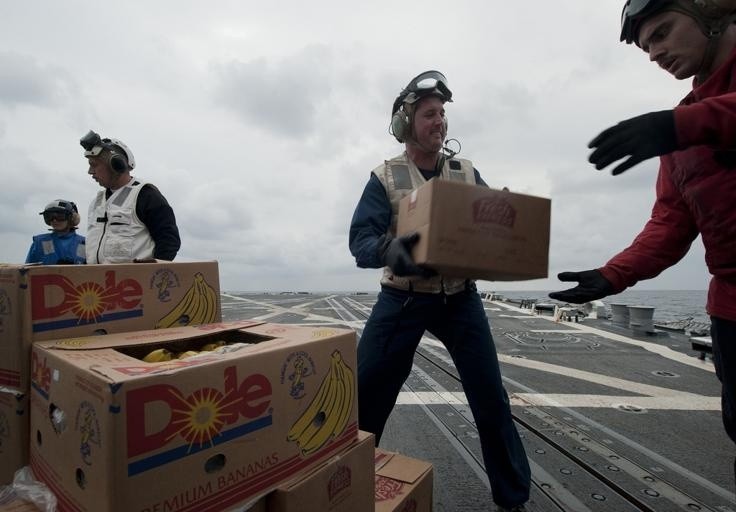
[589,110,680,175]
[549,270,614,304]
[384,233,438,278]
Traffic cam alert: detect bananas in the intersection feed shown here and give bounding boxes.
[142,338,229,362]
[156,270,220,329]
[290,347,354,455]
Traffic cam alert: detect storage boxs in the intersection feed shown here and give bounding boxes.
[392,177,552,284]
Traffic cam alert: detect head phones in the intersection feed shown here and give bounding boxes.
[390,111,414,144]
[108,152,129,177]
[69,212,83,225]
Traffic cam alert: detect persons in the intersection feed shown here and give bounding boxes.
[24,199,87,265]
[345,66,532,512]
[79,129,181,266]
[546,1,736,449]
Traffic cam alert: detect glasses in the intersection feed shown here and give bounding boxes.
[620,0,649,41]
[80,131,98,148]
[408,71,451,96]
[46,212,66,221]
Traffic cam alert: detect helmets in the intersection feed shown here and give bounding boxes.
[84,138,135,174]
[389,88,444,142]
[39,199,80,225]
[620,0,736,44]
